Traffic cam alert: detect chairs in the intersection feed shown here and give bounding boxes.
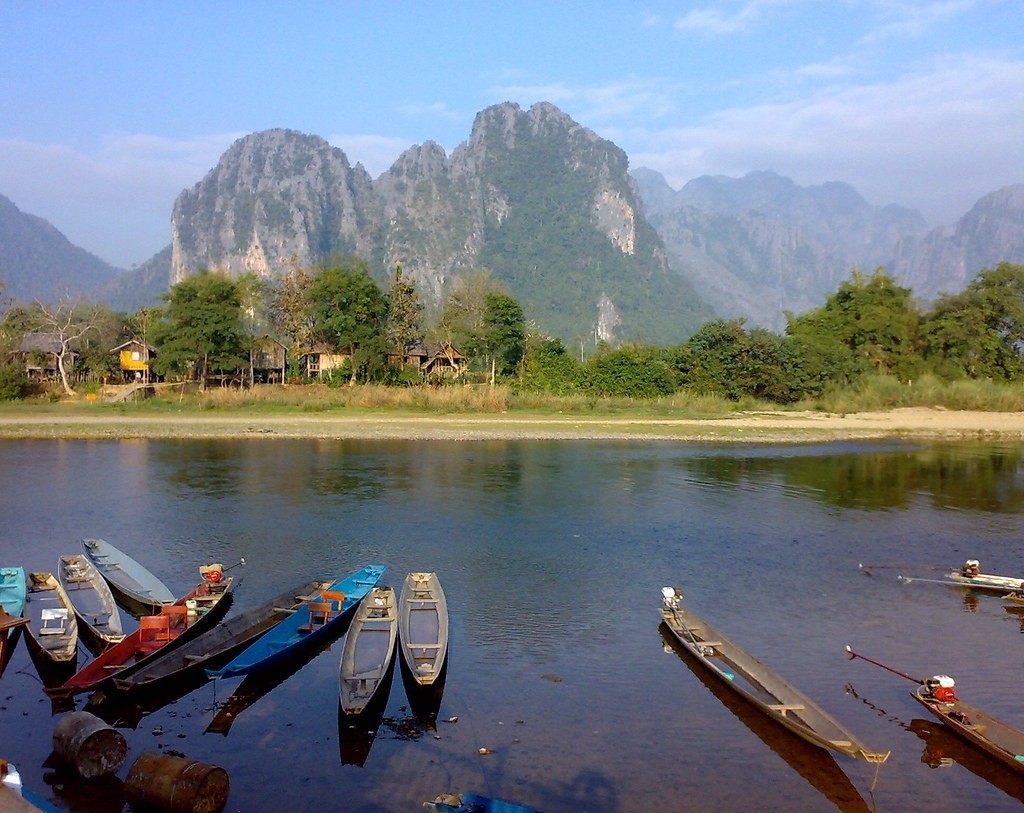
[39,608,69,634]
[135,616,170,651]
[313,591,344,619]
[297,602,330,631]
[155,607,188,641]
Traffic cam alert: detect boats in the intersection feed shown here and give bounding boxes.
[208,565,388,679]
[114,578,336,688]
[656,587,892,762]
[907,669,1024,774]
[42,564,235,702]
[337,584,399,717]
[1,567,26,649]
[57,556,124,644]
[22,572,79,661]
[944,560,1024,590]
[398,571,448,684]
[80,540,174,606]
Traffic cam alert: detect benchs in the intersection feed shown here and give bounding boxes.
[407,643,442,652]
[0,553,119,604]
[367,605,392,614]
[766,704,805,717]
[186,655,203,661]
[689,641,721,651]
[194,596,220,601]
[345,677,381,680]
[664,614,682,619]
[268,640,295,646]
[82,612,111,623]
[412,587,432,596]
[146,674,159,679]
[407,598,439,607]
[353,579,376,585]
[358,618,394,622]
[272,595,311,613]
[343,593,361,600]
[831,740,851,747]
[672,626,699,630]
